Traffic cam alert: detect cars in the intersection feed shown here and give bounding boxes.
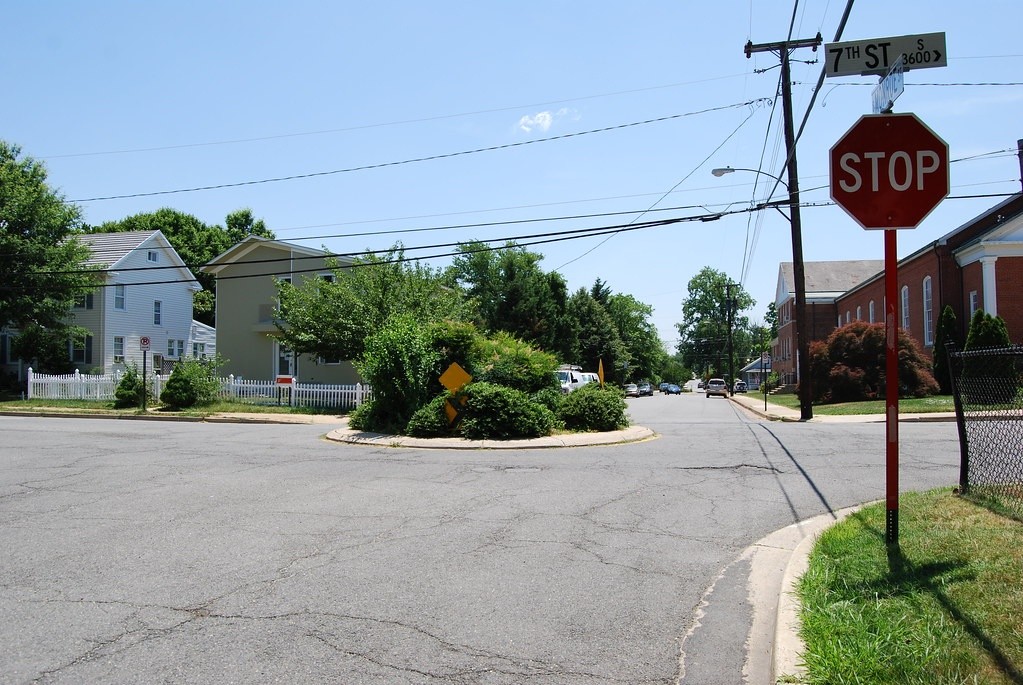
[706,378,728,398]
[659,384,681,395]
[623,384,640,398]
[698,382,706,390]
[639,386,653,396]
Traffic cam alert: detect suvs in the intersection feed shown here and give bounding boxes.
[733,382,748,394]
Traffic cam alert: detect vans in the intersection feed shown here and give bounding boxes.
[554,370,584,394]
[580,373,600,384]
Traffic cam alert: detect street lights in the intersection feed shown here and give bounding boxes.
[711,167,813,420]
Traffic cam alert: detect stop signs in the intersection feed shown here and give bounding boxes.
[827,113,951,230]
